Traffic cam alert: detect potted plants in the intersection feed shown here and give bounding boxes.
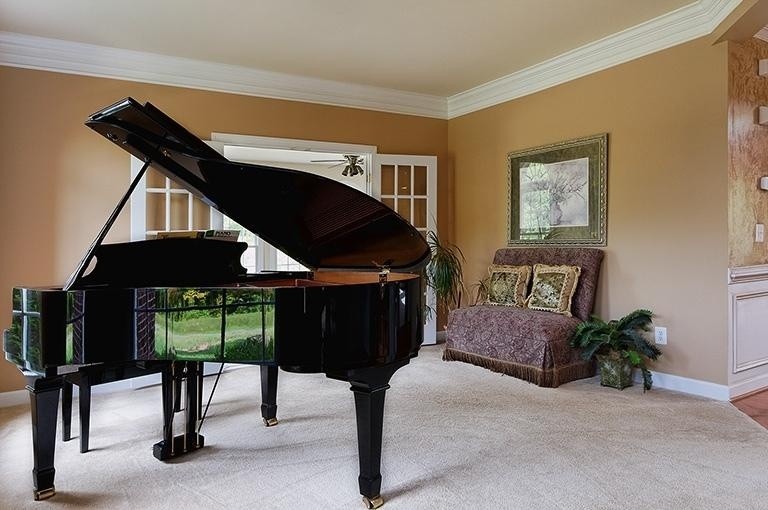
[569,308,662,393]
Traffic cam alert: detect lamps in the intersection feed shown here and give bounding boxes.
[343,164,364,176]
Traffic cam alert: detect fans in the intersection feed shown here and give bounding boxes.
[308,154,365,168]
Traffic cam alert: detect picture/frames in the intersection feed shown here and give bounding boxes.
[505,133,609,246]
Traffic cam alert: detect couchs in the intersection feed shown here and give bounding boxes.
[441,246,604,389]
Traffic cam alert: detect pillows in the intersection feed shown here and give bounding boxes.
[477,263,584,318]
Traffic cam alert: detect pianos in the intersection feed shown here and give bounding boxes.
[4,97,431,509]
